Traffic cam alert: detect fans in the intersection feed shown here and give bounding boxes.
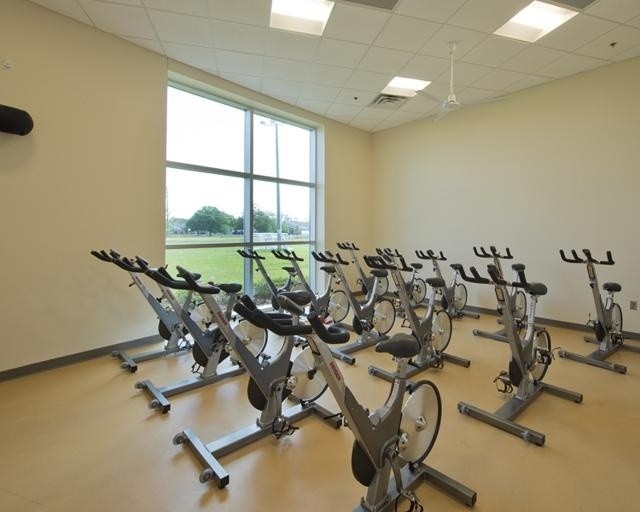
[412,40,510,126]
